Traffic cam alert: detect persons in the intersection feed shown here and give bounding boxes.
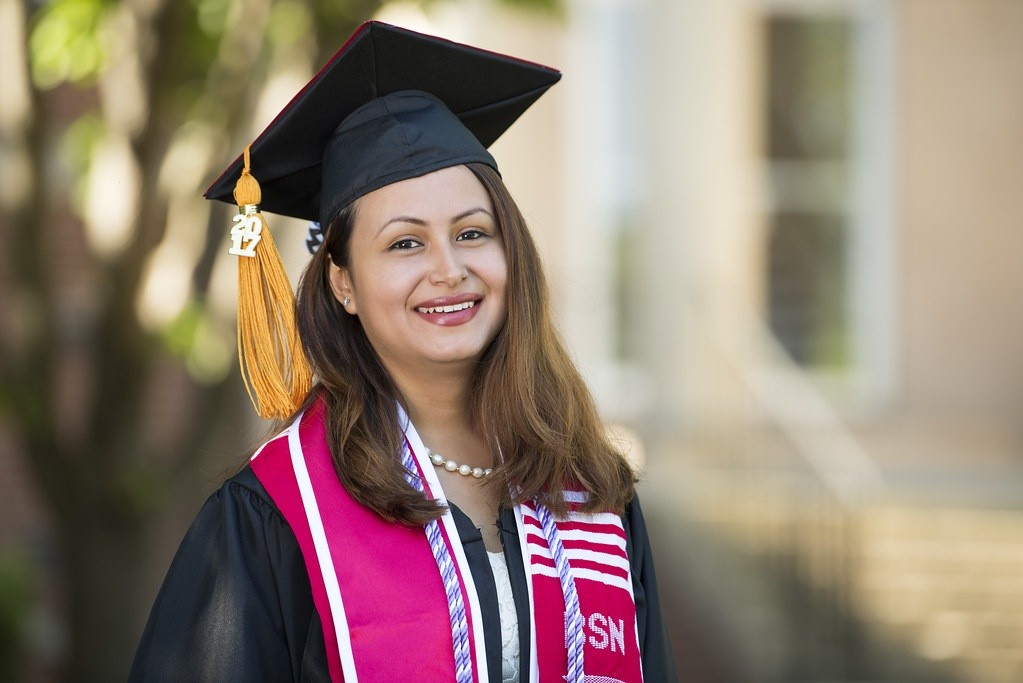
[128,20,679,683]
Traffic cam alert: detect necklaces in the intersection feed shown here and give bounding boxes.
[424,447,505,479]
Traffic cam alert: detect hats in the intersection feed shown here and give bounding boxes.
[200,20,564,421]
